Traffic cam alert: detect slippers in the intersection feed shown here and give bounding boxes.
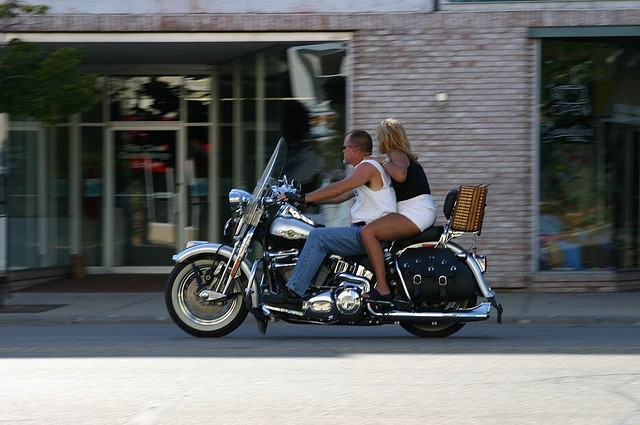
[364,288,392,303]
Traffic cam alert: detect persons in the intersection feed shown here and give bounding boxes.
[361,118,438,300]
[261,131,397,311]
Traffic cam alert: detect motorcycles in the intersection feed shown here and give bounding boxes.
[164,137,503,337]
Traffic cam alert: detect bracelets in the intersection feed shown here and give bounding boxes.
[380,156,390,166]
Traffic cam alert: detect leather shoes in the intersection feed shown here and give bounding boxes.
[262,288,303,309]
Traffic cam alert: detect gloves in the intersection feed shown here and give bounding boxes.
[284,191,305,202]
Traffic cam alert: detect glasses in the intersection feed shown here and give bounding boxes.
[341,145,354,148]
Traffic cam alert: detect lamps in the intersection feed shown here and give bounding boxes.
[435,93,449,104]
